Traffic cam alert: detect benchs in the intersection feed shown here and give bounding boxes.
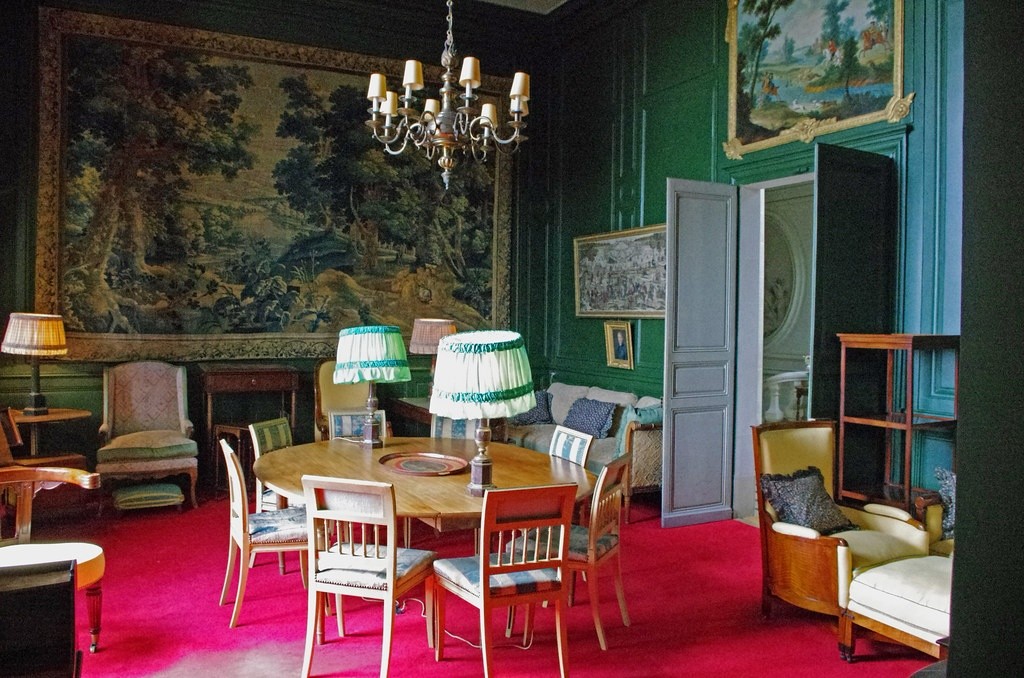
[500,381,665,526]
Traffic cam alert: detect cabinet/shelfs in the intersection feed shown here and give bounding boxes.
[835,333,960,528]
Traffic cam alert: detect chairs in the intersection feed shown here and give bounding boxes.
[248,416,307,568]
[218,439,332,628]
[750,417,931,661]
[300,474,439,678]
[0,465,106,654]
[0,559,85,678]
[915,464,955,558]
[0,421,90,526]
[327,409,388,440]
[95,359,198,519]
[430,413,490,440]
[431,481,578,678]
[505,453,632,651]
[548,425,594,469]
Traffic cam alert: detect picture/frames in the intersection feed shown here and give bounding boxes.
[721,0,916,160]
[572,224,667,320]
[36,5,515,365]
[603,320,635,370]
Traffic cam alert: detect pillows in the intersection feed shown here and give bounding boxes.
[512,390,557,427]
[933,465,957,541]
[562,398,618,439]
[613,403,664,461]
[758,465,862,539]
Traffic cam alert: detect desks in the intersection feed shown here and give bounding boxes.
[12,407,92,459]
[254,435,597,556]
[197,362,299,490]
[391,396,432,438]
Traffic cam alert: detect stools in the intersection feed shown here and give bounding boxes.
[111,483,185,520]
[845,553,953,662]
[212,419,264,505]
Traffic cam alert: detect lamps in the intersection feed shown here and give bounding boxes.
[1,312,68,415]
[410,318,457,404]
[332,326,412,450]
[365,0,530,188]
[429,330,537,496]
